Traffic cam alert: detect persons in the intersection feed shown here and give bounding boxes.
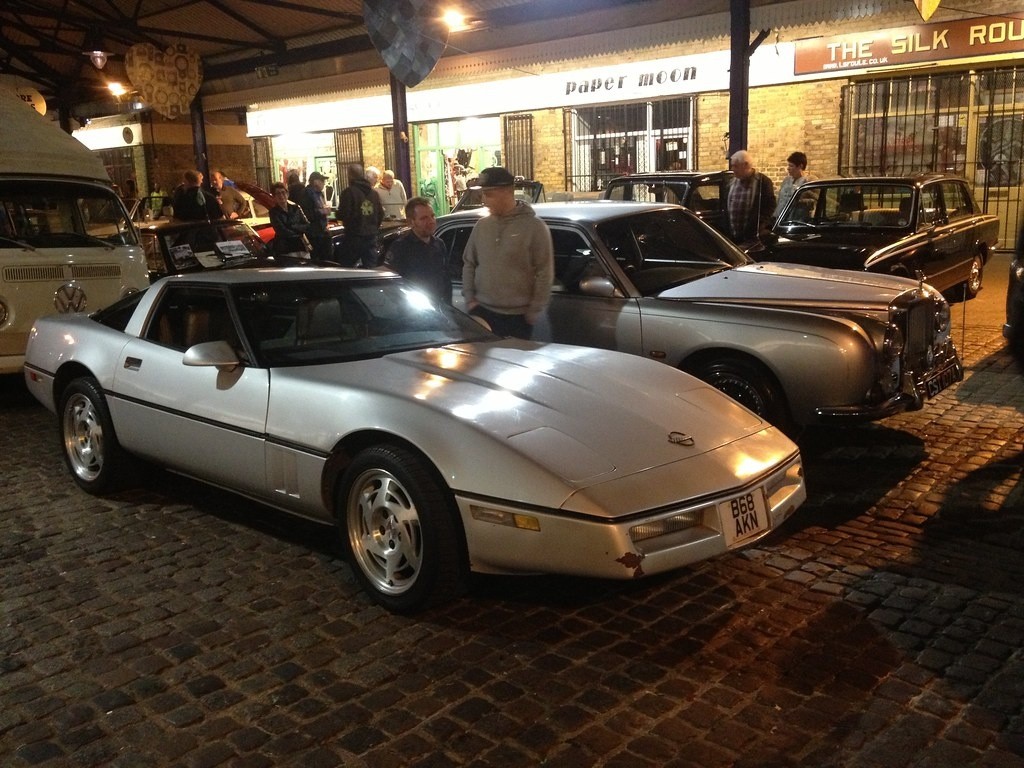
[725,150,777,244]
[151,169,247,220]
[270,164,407,269]
[771,152,820,222]
[383,197,452,304]
[462,167,555,340]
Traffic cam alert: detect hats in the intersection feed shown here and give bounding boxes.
[466,167,515,187]
[308,171,329,181]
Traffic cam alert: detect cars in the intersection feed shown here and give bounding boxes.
[24,267,807,617]
[392,200,965,464]
[753,173,1000,299]
[604,171,738,241]
[120,176,546,286]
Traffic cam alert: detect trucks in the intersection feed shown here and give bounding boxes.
[0,85,149,381]
